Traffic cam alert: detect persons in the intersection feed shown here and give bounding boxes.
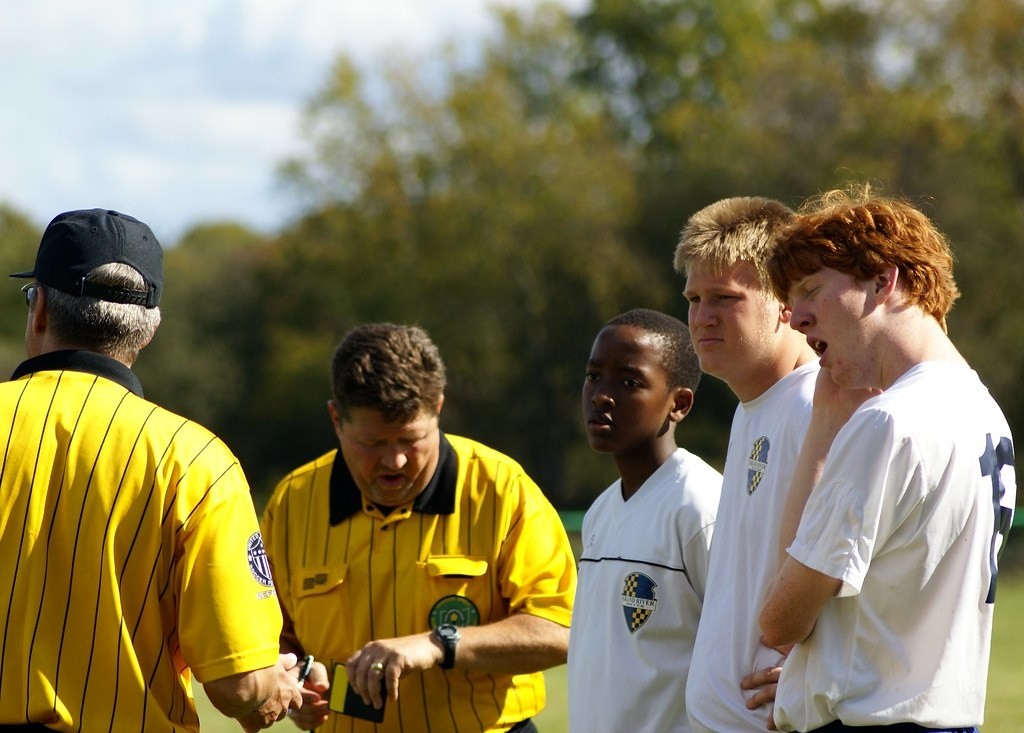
[260,323,578,733]
[673,195,823,733]
[0,207,302,733]
[760,178,1016,733]
[568,310,724,733]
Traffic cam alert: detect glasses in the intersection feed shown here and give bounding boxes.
[21,282,35,304]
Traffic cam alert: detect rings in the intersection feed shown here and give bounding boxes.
[370,662,385,671]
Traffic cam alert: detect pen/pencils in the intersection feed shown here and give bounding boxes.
[287,653,315,719]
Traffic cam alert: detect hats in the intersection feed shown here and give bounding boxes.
[9,208,163,307]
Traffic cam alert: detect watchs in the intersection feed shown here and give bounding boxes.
[433,624,461,670]
